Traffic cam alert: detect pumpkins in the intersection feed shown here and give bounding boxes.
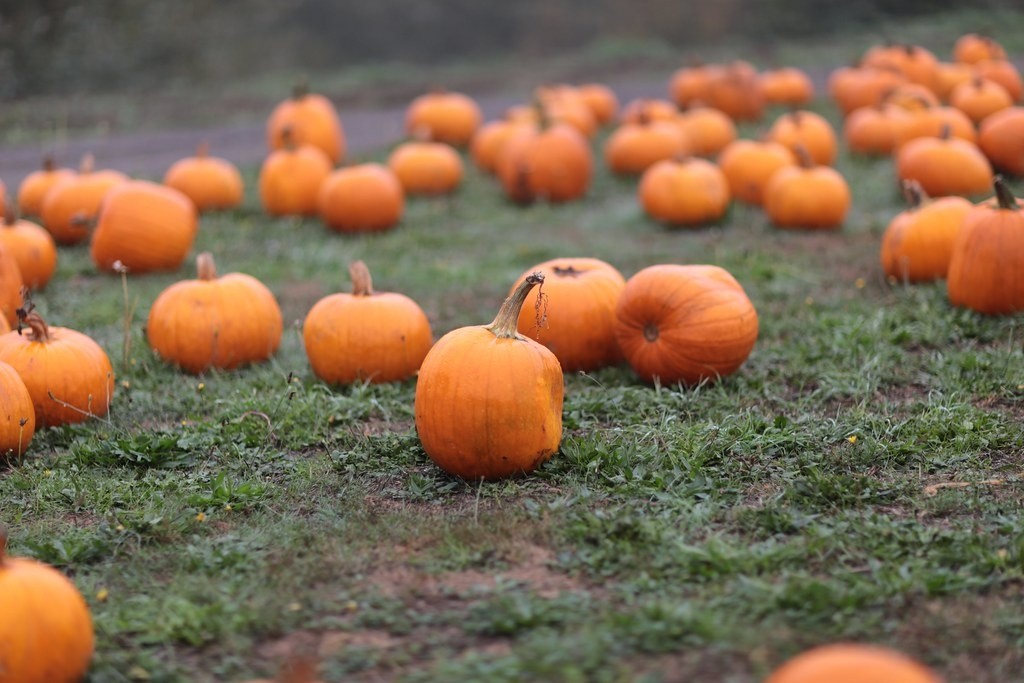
[1,35,1023,478]
[768,644,939,682]
[0,529,94,683]
